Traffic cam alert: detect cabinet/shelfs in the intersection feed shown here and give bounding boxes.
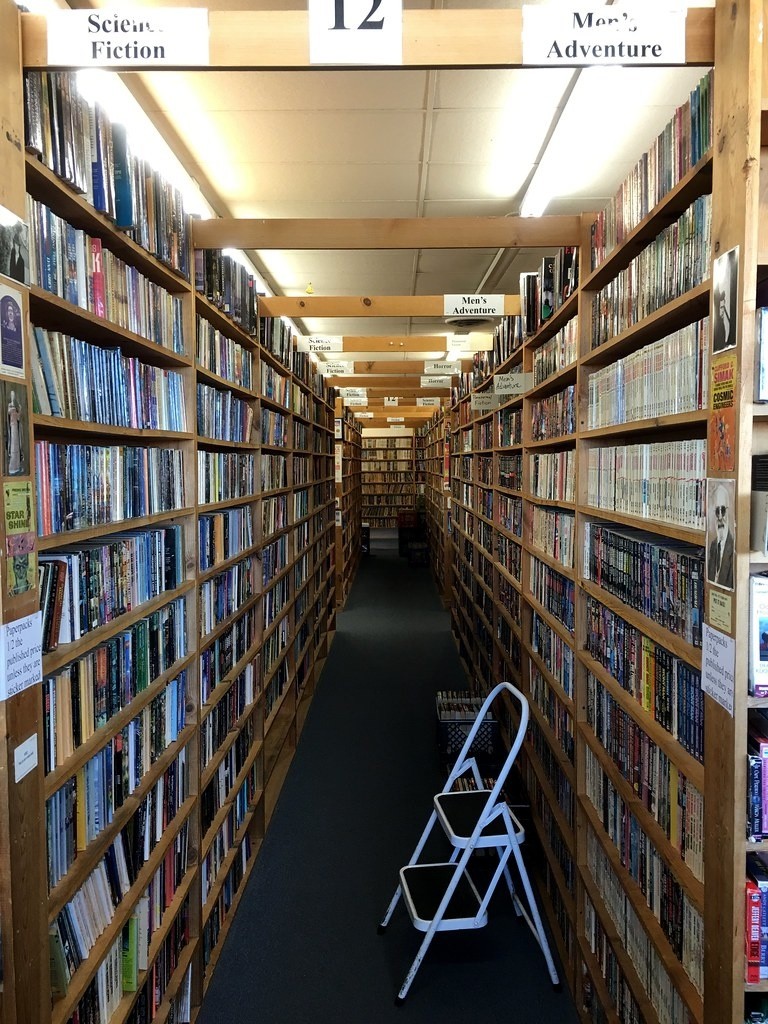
[0,0,768,1024]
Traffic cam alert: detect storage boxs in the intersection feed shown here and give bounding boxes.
[396,512,415,527]
[361,522,370,554]
[433,695,500,765]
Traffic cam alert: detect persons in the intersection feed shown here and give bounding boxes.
[713,290,734,345]
[7,233,28,285]
[3,302,21,334]
[701,484,734,588]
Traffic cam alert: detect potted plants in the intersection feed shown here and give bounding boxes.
[416,495,425,525]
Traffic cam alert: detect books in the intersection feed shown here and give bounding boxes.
[360,437,415,527]
[3,71,359,1021]
[415,66,768,1023]
